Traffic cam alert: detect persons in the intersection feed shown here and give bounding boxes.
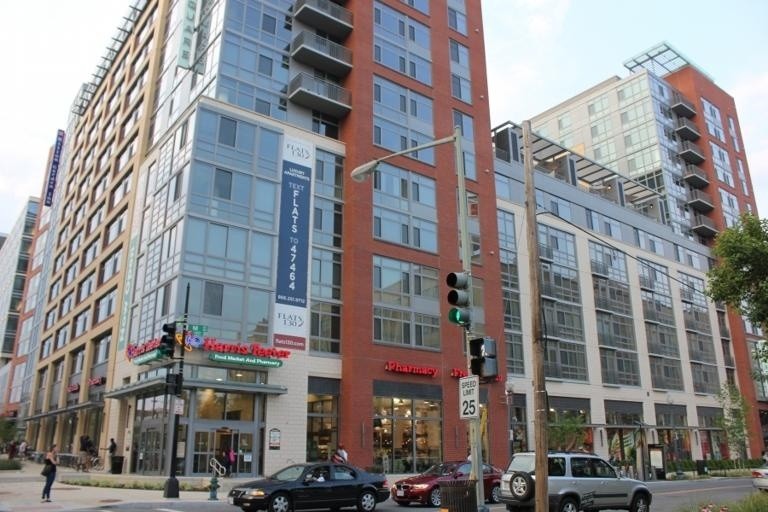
[76,435,94,472]
[2,440,33,460]
[218,438,232,478]
[100,438,117,472]
[40,444,60,503]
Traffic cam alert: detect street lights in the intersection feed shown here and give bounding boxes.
[349,124,491,512]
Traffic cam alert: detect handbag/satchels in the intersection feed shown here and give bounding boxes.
[41,465,52,475]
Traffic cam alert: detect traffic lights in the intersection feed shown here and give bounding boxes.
[160,321,176,356]
[446,271,471,327]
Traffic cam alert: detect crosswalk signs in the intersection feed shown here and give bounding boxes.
[468,336,497,380]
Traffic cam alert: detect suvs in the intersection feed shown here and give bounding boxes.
[498,450,656,512]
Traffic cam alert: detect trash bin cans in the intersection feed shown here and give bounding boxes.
[655,468,665,480]
[437,480,479,512]
[111,456,124,473]
[697,460,707,475]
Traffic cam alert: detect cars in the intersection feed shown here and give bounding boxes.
[391,459,504,508]
[227,461,391,512]
